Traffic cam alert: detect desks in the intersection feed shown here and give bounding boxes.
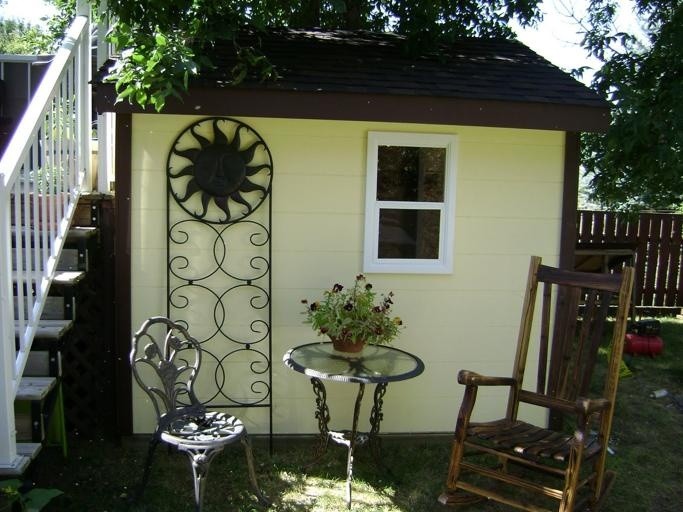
[283,341,425,510]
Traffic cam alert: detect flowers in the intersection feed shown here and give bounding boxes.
[299,271,406,346]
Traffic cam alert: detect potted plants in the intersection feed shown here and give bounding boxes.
[26,158,70,233]
[38,94,100,191]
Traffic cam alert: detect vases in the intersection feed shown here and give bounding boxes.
[331,335,368,353]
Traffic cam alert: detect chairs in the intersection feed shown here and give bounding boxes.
[438,255,636,512]
[127,315,273,512]
